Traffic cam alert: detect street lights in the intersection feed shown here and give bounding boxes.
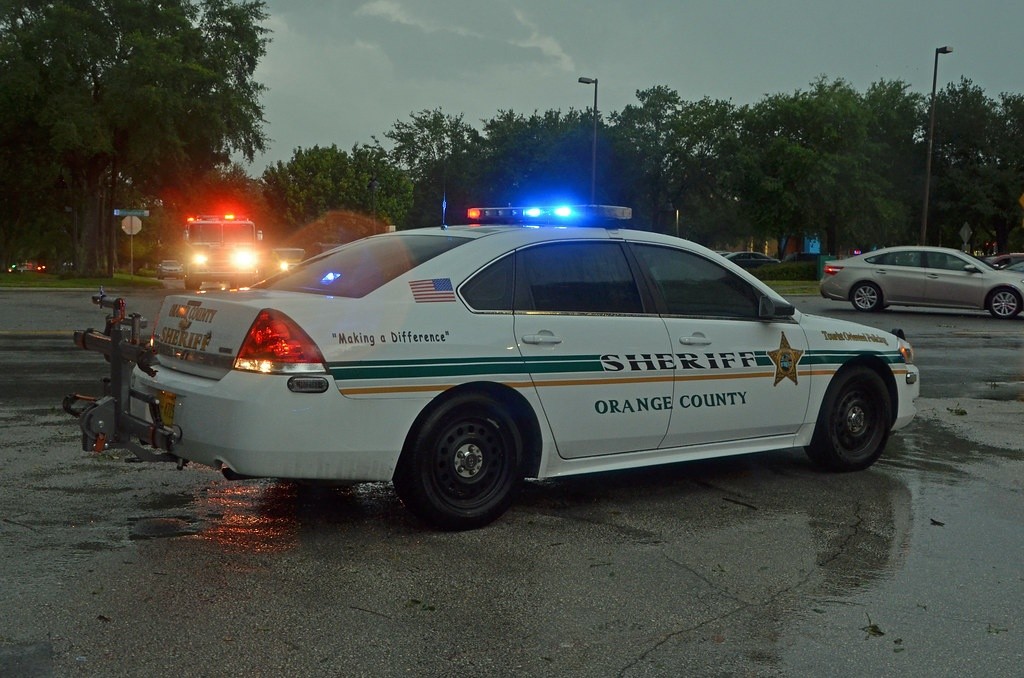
[578,77,599,205]
[920,46,953,246]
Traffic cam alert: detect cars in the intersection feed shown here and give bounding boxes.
[783,253,819,261]
[820,246,1024,320]
[724,252,781,269]
[976,253,1024,273]
[156,260,184,280]
[130,205,921,532]
[268,244,341,274]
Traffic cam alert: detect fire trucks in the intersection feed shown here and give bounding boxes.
[182,214,263,291]
